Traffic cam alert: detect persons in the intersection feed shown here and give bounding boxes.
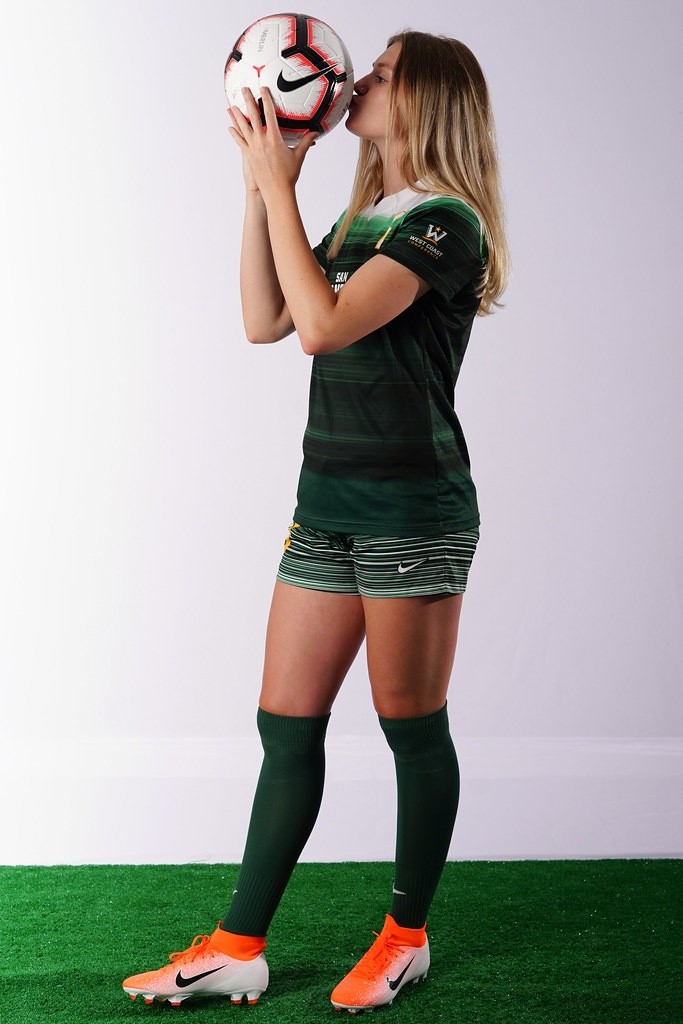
[119,33,508,1010]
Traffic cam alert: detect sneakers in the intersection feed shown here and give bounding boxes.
[329,914,431,1013]
[121,934,270,1005]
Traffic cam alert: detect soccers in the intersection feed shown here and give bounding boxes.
[223,13,355,149]
